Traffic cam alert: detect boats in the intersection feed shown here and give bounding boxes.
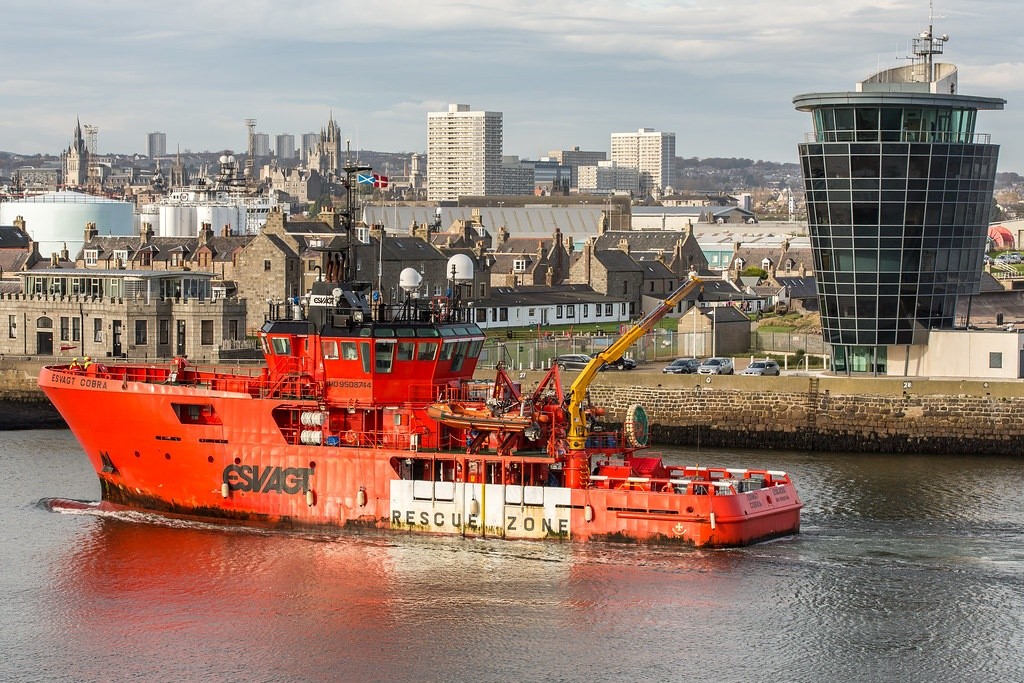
[34,138,806,549]
[427,401,549,431]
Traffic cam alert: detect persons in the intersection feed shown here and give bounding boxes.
[755,308,763,322]
[69,358,82,370]
[593,462,600,475]
[84,357,93,369]
[177,355,192,379]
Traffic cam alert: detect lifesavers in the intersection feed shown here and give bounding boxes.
[104,374,111,380]
[345,430,356,442]
[661,484,674,494]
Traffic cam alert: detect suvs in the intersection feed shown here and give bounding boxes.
[740,360,780,376]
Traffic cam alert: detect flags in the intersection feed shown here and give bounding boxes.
[374,174,388,187]
[358,174,375,184]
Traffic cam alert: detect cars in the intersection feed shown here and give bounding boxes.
[995,252,1024,264]
[697,358,734,375]
[663,358,701,374]
[590,352,637,371]
[557,355,591,371]
